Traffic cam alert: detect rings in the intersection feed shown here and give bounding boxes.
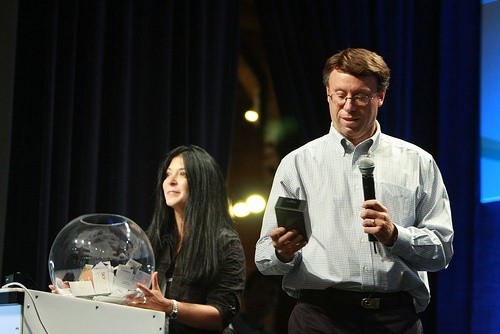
[143,296,147,304]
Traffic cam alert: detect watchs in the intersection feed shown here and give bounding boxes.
[168,299,178,320]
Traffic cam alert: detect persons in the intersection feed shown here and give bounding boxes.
[255,48,454,334]
[49,145,246,334]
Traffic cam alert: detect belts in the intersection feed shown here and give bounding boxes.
[299,288,412,311]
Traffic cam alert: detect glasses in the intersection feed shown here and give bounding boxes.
[328,92,380,106]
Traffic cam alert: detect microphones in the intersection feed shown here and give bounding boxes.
[358,158,378,242]
